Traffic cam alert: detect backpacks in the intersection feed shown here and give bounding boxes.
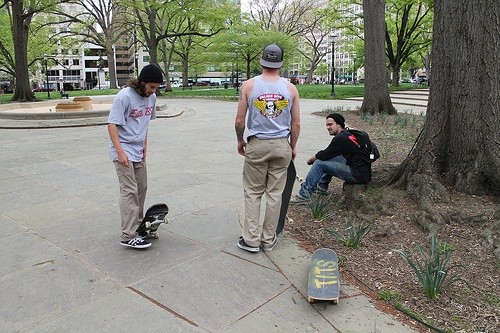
[343,125,379,164]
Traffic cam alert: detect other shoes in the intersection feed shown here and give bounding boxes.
[261,236,278,251]
[237,239,259,253]
[289,194,311,205]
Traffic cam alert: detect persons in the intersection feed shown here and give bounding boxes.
[107,64,163,248]
[289,77,303,85]
[289,113,372,204]
[235,43,300,252]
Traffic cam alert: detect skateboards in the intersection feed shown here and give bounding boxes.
[307,248,340,305]
[275,158,297,237]
[135,202,170,240]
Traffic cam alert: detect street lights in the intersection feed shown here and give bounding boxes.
[44,54,52,98]
[235,44,239,93]
[329,31,337,96]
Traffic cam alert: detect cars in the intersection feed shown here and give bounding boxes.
[401,78,411,83]
[159,80,167,89]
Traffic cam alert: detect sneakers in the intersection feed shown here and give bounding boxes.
[120,237,152,249]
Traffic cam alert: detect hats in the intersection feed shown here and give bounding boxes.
[259,43,284,69]
